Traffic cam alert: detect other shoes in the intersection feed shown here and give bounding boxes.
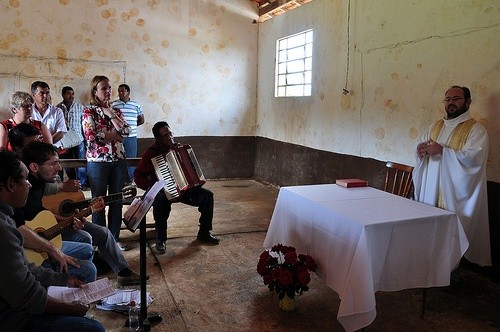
[197,230,220,245]
[117,270,150,285]
[156,241,166,254]
[115,239,129,250]
[132,182,136,186]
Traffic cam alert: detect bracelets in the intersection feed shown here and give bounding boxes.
[109,114,117,118]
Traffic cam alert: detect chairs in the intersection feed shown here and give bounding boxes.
[383,161,417,199]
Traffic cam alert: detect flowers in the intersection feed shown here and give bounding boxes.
[257,243,318,300]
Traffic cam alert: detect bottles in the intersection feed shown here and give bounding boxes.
[128,301,139,332]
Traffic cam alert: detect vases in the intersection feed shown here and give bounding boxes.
[279,294,295,310]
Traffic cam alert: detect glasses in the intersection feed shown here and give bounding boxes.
[444,97,464,101]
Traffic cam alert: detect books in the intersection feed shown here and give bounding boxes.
[335,178,367,189]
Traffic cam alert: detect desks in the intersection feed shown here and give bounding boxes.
[262,183,469,332]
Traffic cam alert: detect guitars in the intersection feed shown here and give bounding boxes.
[41,184,138,222]
[23,195,105,267]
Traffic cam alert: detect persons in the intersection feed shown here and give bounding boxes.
[81,75,131,251]
[111,83,144,187]
[56,86,87,191]
[134,121,221,254]
[412,85,491,297]
[0,146,106,332]
[0,124,150,332]
[0,81,68,153]
